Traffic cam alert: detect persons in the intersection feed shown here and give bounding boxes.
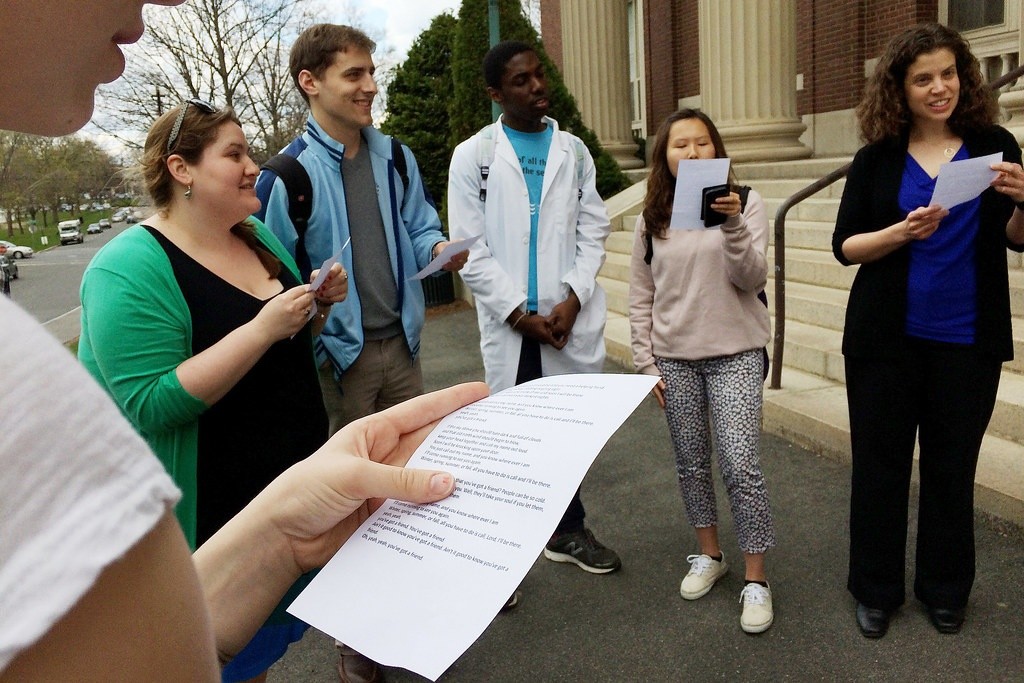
[76,97,337,682]
[447,39,620,611]
[831,25,1024,640]
[0,0,227,683]
[630,107,775,634]
[251,24,470,683]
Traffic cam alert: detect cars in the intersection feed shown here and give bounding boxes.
[0,240,33,259]
[0,256,18,280]
[61,203,111,212]
[87,223,103,234]
[99,219,112,229]
[127,215,137,224]
[112,206,144,222]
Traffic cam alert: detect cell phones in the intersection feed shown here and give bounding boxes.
[705,187,729,228]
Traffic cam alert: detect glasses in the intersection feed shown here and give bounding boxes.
[166,98,222,157]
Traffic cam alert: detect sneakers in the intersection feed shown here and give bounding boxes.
[544,528,621,573]
[681,551,728,600]
[740,580,774,633]
[500,592,517,610]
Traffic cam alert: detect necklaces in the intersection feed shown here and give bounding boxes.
[926,134,955,159]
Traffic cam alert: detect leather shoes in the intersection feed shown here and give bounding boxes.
[338,653,377,683]
[856,602,889,637]
[929,604,960,632]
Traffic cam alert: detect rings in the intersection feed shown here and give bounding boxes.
[343,273,348,279]
[305,308,312,317]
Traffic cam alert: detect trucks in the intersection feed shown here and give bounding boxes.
[57,220,83,246]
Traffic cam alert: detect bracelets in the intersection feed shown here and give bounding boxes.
[512,310,530,330]
